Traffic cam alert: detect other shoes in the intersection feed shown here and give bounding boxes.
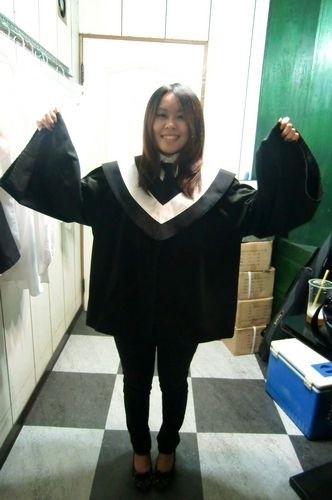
[133,453,153,490]
[152,451,176,493]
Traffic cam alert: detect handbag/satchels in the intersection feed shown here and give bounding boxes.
[311,297,332,345]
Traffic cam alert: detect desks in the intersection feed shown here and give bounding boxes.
[281,315,331,364]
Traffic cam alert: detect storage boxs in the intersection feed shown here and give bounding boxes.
[222,326,266,356]
[263,338,332,441]
[238,268,275,301]
[237,298,274,328]
[239,241,274,272]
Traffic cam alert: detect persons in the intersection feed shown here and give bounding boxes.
[0,82,323,493]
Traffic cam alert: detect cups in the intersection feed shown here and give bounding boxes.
[306,279,332,327]
